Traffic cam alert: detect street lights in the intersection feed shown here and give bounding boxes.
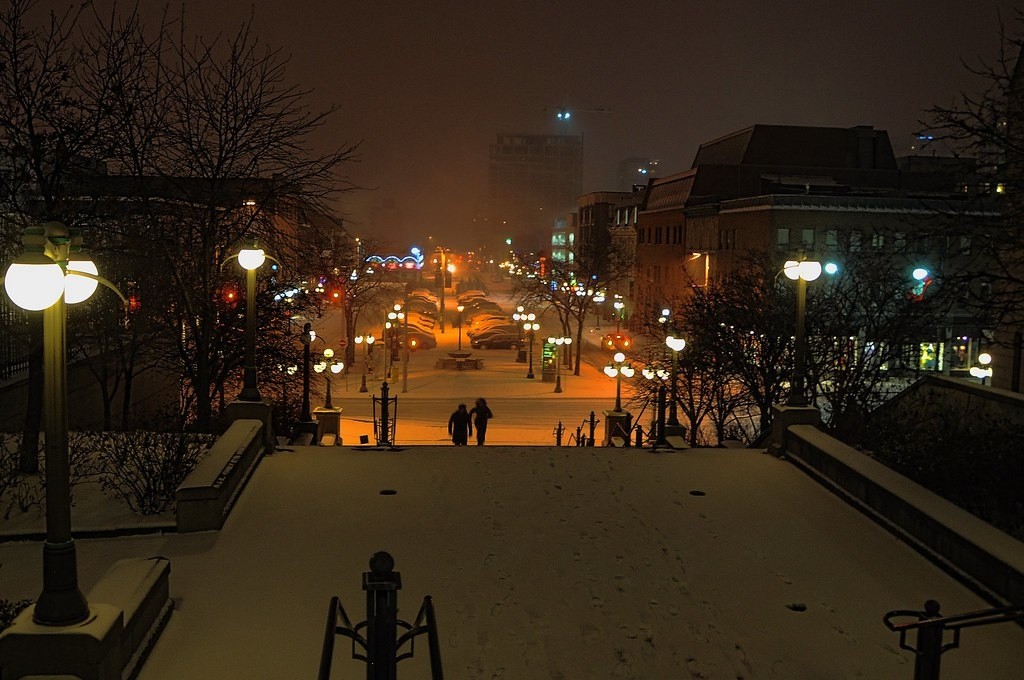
[219,246,285,401]
[313,348,344,409]
[614,295,625,334]
[355,332,376,393]
[1,219,131,631]
[642,363,670,443]
[456,304,465,351]
[665,328,686,426]
[592,291,605,330]
[773,247,822,407]
[287,322,326,423]
[385,303,405,362]
[548,333,573,393]
[523,312,541,379]
[603,352,636,413]
[512,305,527,362]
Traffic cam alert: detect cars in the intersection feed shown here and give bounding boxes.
[600,333,632,354]
[382,287,438,350]
[458,290,526,351]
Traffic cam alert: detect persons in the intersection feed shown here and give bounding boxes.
[449,404,472,445]
[469,398,493,446]
